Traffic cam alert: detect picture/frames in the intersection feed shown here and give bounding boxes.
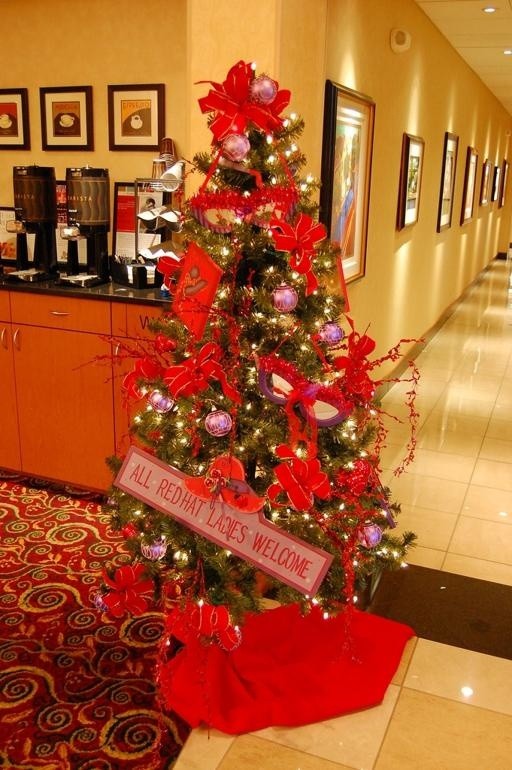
[394,131,509,233]
[108,84,165,152]
[318,79,375,283]
[0,88,31,150]
[40,86,94,152]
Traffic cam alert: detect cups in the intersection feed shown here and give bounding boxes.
[61,114,75,124]
[131,115,140,125]
[149,137,184,192]
[1,114,10,124]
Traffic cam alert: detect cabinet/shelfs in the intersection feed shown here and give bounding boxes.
[1,290,112,495]
[111,304,174,463]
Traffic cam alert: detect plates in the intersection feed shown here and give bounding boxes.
[59,121,74,128]
[0,121,12,129]
[131,120,143,129]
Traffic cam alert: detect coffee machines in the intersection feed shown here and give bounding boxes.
[58,167,112,289]
[7,166,59,283]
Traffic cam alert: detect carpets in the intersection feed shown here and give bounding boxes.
[1,469,192,769]
